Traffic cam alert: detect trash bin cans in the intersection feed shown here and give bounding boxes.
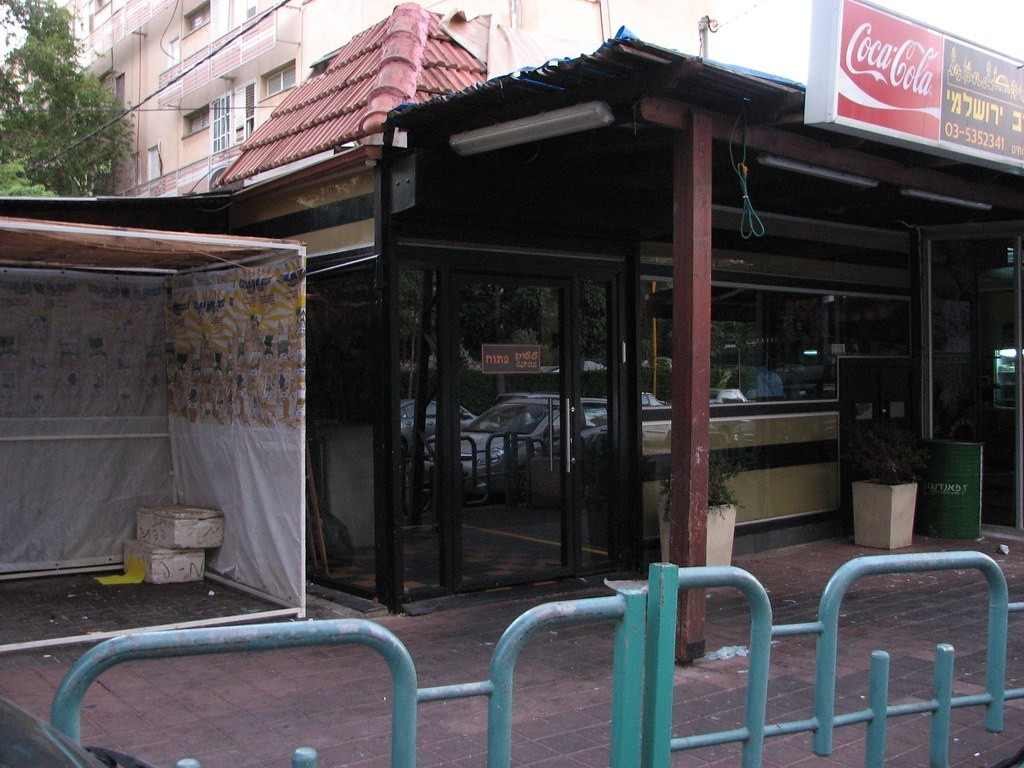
[918,439,983,538]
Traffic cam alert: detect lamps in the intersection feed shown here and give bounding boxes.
[756,154,881,191]
[901,184,994,214]
[444,100,617,159]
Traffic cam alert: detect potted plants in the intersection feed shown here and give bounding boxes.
[656,445,748,568]
[842,416,928,552]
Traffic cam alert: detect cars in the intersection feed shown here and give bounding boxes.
[401,398,499,454]
[543,357,673,371]
[494,388,758,406]
[407,397,608,501]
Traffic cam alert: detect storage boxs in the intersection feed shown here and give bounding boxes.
[134,501,226,549]
[119,538,210,586]
